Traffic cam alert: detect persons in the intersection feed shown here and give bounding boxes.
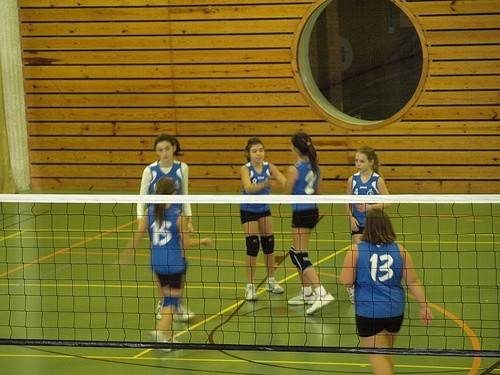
[284,132,335,313]
[137,134,196,323]
[116,179,212,353]
[340,208,434,375]
[346,146,390,303]
[239,137,285,300]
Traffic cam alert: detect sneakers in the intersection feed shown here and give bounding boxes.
[305,292,335,314]
[288,292,316,304]
[154,333,181,352]
[155,301,162,319]
[245,285,258,301]
[265,280,284,293]
[173,306,196,321]
[345,285,355,304]
